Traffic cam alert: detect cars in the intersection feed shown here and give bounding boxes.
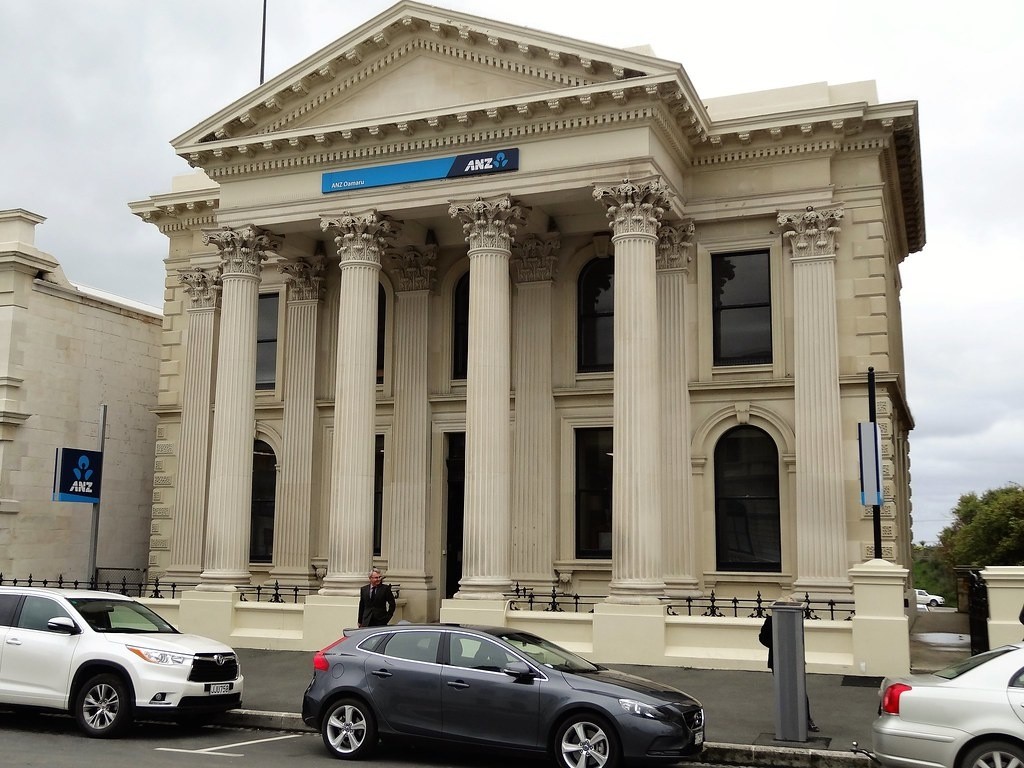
[0,585,244,738]
[915,589,945,608]
[871,635,1024,768]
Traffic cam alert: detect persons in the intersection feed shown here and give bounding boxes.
[759,614,820,733]
[358,568,396,628]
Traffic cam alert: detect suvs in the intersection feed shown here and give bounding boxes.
[301,624,707,768]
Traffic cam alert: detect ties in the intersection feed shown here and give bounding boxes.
[371,587,375,598]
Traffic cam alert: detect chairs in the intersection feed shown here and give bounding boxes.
[489,647,508,663]
[80,610,111,628]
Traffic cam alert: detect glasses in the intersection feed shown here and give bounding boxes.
[371,576,382,579]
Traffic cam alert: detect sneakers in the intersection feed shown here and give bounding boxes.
[807,719,820,732]
